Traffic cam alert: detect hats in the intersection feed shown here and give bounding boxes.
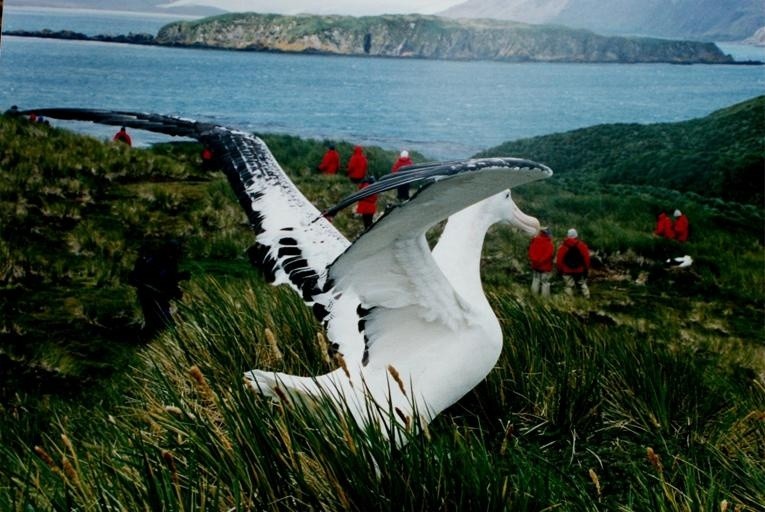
[567,228,578,238]
[354,146,362,152]
[328,144,336,150]
[674,209,681,216]
[400,150,409,158]
[369,175,375,183]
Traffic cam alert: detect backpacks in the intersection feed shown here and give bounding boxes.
[562,240,582,269]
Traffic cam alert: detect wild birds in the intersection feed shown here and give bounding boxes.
[11,103,555,481]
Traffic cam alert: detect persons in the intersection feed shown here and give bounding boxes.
[357,176,377,230]
[651,207,676,240]
[392,150,414,200]
[3,105,51,128]
[346,145,368,185]
[672,207,689,242]
[556,228,591,300]
[315,144,341,176]
[113,128,131,146]
[528,228,554,298]
